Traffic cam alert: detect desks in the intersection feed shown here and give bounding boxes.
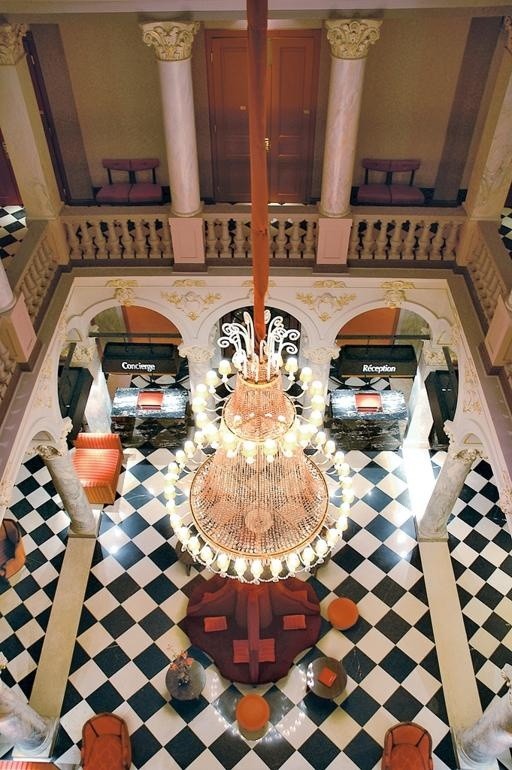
[236,694,270,732]
[165,659,206,703]
[305,657,347,700]
[326,598,358,632]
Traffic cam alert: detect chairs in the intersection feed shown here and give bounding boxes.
[381,720,435,770]
[357,159,392,207]
[390,159,426,206]
[94,159,131,207]
[80,712,133,770]
[1,518,27,579]
[128,158,162,206]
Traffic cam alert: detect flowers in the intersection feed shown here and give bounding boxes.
[168,651,194,685]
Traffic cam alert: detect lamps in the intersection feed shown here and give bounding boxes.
[163,2,356,584]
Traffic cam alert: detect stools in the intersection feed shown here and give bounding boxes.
[259,638,277,663]
[233,639,250,665]
[283,614,307,631]
[292,590,308,603]
[203,615,228,633]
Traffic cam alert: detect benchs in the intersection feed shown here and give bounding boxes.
[71,432,127,506]
